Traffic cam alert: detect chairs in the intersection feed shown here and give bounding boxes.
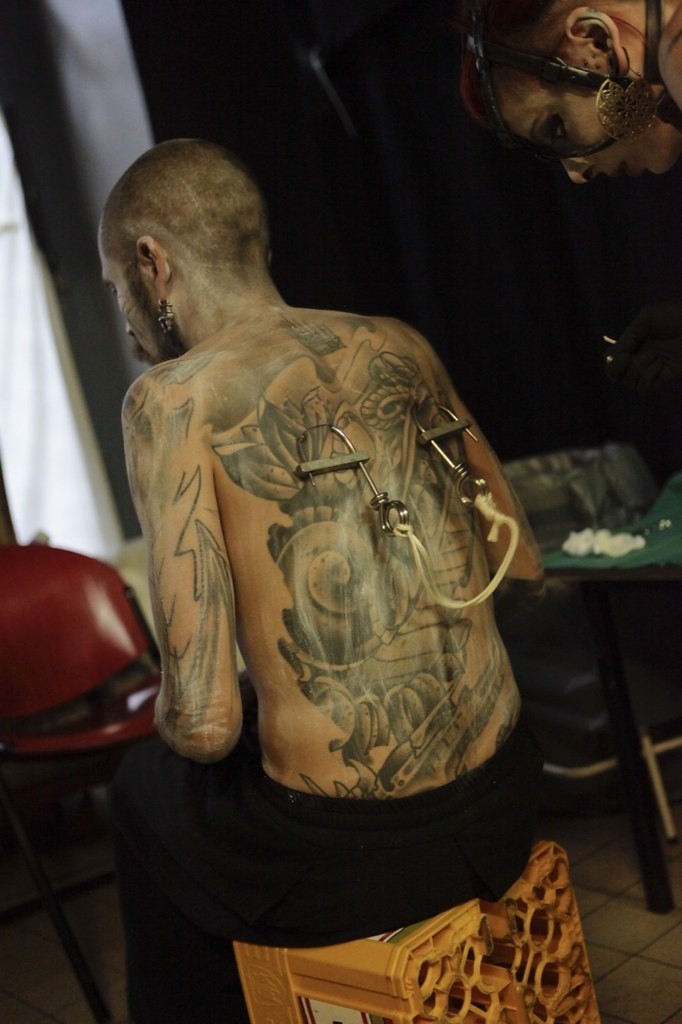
[501,446,682,846]
[1,543,164,1024]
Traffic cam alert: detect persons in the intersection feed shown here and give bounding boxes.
[94,138,557,1021]
[460,1,681,183]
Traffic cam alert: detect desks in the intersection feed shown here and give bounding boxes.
[491,519,682,916]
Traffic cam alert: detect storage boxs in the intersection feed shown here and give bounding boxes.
[232,838,601,1024]
